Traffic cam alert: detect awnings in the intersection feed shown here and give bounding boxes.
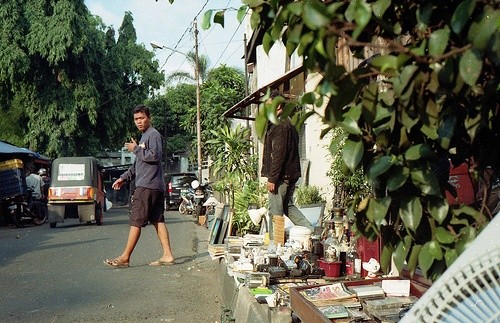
[221,65,307,121]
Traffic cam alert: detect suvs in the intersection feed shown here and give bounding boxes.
[163,172,198,211]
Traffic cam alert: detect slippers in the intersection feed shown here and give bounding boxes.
[149,258,175,265]
[102,256,130,267]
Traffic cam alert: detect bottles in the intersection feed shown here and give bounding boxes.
[324,206,361,278]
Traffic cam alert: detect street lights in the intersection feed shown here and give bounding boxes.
[149,40,202,185]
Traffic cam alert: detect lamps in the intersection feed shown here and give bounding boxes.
[202,194,218,215]
[246,207,270,246]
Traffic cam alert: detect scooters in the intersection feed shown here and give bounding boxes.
[176,177,214,215]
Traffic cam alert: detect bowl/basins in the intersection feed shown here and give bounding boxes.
[290,226,311,243]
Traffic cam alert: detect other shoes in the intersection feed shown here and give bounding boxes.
[45,220,48,222]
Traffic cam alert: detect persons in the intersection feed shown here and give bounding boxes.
[25,168,51,200]
[261,91,314,240]
[103,106,175,268]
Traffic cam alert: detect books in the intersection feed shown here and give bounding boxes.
[206,236,261,260]
[299,282,362,319]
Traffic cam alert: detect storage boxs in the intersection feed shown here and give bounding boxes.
[0,159,27,198]
[289,277,424,323]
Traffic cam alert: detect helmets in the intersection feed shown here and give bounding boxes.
[38,168,46,176]
[191,179,200,189]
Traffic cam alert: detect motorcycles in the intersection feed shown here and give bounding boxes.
[2,184,48,230]
[47,157,107,228]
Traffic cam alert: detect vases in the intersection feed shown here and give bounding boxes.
[317,259,342,277]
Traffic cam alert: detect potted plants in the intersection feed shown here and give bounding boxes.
[296,186,325,226]
[211,181,227,203]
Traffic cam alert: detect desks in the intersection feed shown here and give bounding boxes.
[217,258,291,323]
